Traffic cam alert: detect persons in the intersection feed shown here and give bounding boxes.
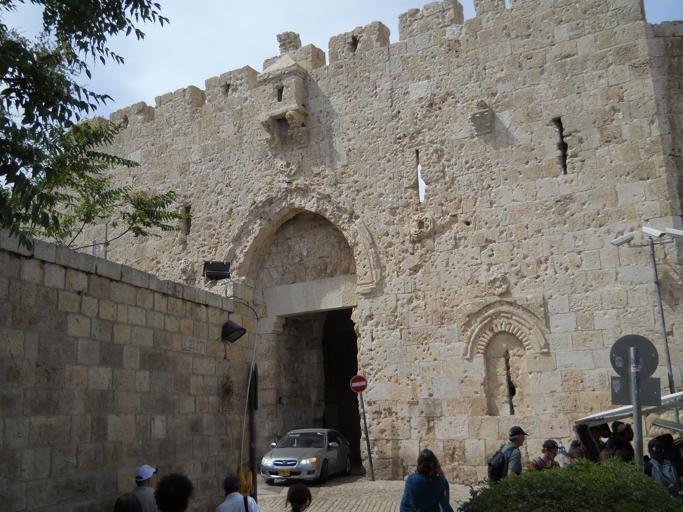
[555,420,682,500]
[398,448,454,512]
[283,482,312,512]
[525,438,561,474]
[486,424,529,484]
[129,463,159,511]
[112,491,144,511]
[153,471,193,511]
[214,472,262,512]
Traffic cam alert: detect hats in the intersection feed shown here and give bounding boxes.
[134,464,156,481]
[223,474,240,490]
[508,426,528,436]
[542,439,558,449]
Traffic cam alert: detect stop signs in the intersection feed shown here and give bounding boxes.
[349,375,367,393]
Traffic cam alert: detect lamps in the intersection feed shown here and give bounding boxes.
[221,319,246,344]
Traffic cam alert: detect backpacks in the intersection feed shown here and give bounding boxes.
[487,443,517,480]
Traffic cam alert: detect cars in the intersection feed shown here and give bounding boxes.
[260,427,352,484]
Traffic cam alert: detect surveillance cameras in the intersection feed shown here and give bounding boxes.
[642,226,666,238]
[666,228,683,236]
[611,233,634,246]
